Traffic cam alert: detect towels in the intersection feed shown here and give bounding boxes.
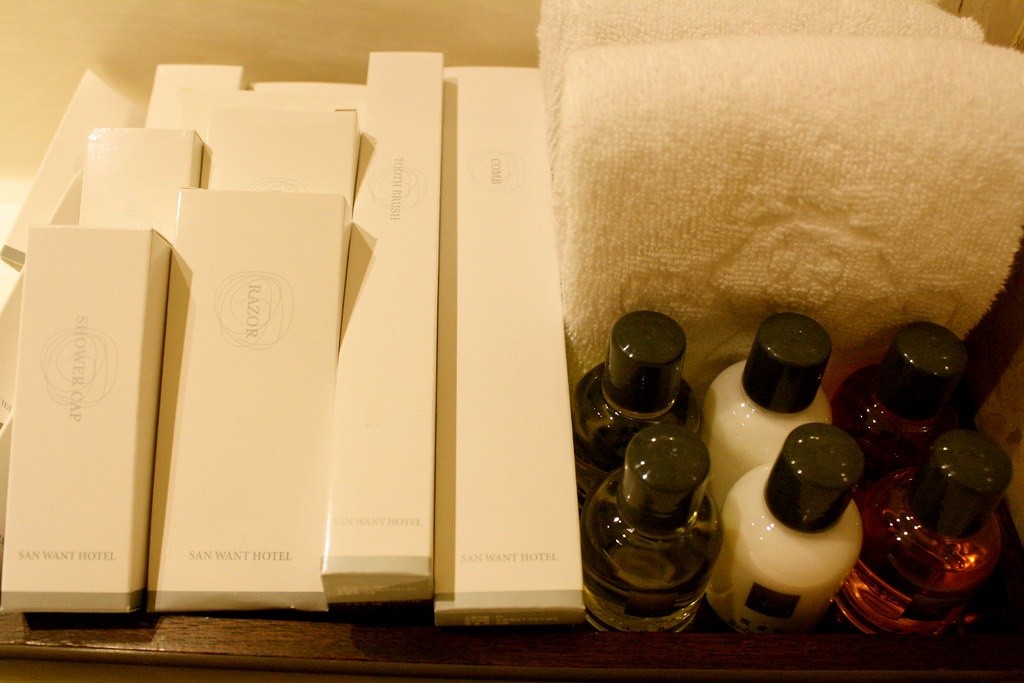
[530,1,1023,455]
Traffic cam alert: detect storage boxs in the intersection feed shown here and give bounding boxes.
[0,50,584,626]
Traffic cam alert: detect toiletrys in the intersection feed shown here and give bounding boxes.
[569,310,1024,634]
